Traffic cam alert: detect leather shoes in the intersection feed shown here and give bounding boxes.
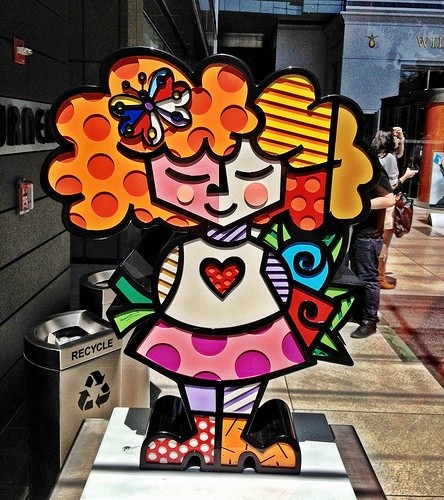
[351,324,377,337]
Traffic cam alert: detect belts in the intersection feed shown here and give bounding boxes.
[358,234,383,240]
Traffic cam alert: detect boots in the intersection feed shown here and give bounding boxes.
[379,257,395,289]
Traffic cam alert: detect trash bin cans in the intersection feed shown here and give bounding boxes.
[78,269,150,411]
[22,309,150,499]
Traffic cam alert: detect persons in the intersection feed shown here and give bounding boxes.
[350,127,418,338]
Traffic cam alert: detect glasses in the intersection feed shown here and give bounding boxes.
[393,134,399,139]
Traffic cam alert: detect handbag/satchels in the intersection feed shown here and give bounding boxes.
[396,179,413,237]
